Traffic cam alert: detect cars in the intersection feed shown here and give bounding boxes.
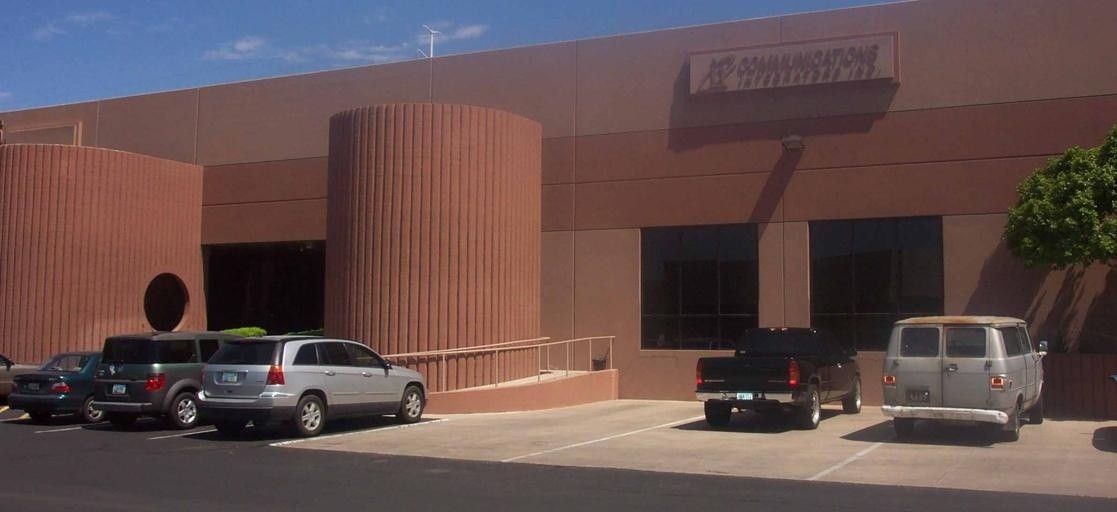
[8,352,105,424]
[0,352,61,401]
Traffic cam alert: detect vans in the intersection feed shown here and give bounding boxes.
[92,332,247,430]
[880,315,1050,441]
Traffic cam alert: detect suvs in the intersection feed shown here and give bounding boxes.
[193,334,430,437]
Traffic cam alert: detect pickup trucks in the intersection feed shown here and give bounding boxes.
[694,326,862,430]
